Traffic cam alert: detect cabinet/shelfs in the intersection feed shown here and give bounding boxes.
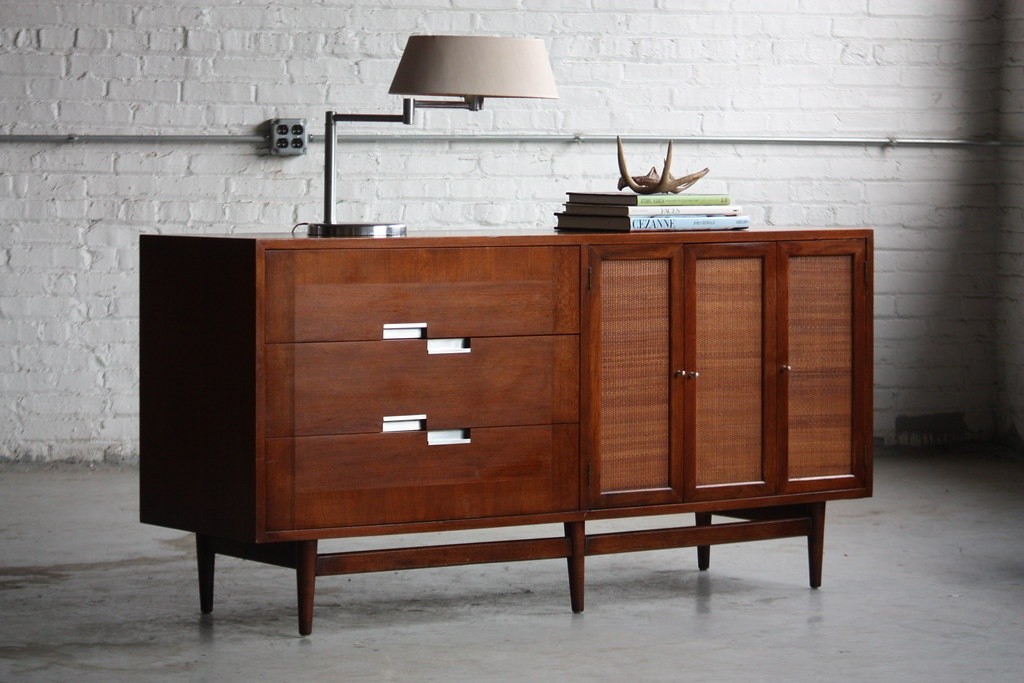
[140,230,875,635]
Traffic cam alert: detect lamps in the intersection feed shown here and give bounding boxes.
[306,36,559,236]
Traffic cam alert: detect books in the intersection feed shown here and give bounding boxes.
[554,192,750,233]
